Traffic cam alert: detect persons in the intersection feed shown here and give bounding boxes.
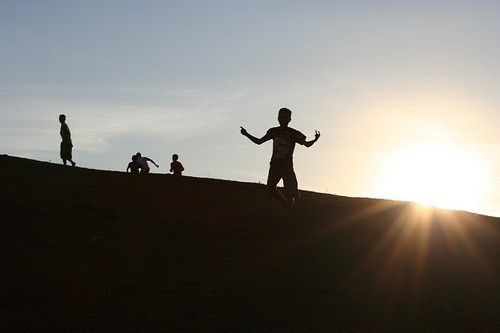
[126,152,159,174]
[58,114,78,167]
[240,107,320,220]
[170,154,184,176]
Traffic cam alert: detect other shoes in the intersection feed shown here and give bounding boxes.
[279,211,296,227]
